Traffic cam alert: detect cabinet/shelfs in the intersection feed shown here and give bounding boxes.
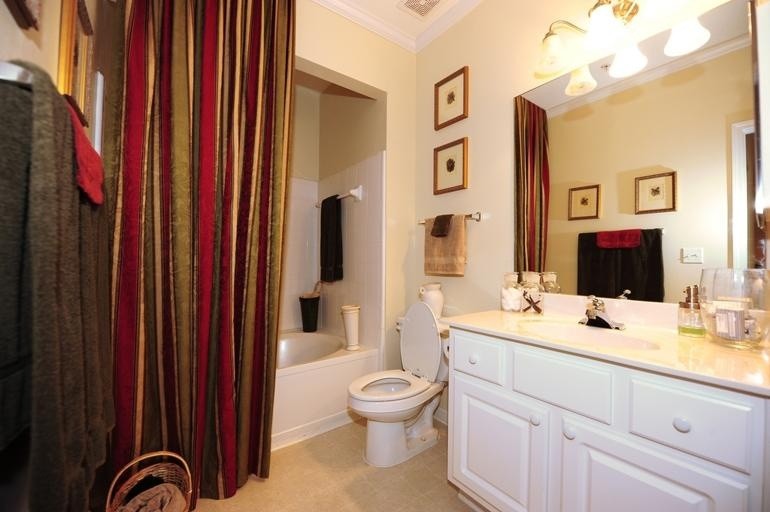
[447,328,769,512]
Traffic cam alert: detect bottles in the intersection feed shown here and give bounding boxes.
[540,272,562,294]
[521,272,544,320]
[518,271,526,286]
[501,270,521,312]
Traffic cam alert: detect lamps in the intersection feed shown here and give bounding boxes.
[534,21,588,81]
[588,1,639,40]
[565,17,710,97]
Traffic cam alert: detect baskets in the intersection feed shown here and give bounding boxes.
[106,451,192,512]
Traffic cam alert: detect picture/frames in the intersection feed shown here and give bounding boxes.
[434,136,468,195]
[568,184,598,221]
[434,66,469,130]
[635,171,677,214]
[57,1,95,128]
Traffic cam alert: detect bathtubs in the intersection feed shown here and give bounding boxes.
[271,326,379,451]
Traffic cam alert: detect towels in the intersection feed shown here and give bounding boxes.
[65,101,105,206]
[122,483,187,512]
[597,228,641,249]
[424,212,467,277]
[321,195,343,282]
[1,59,117,512]
[431,214,453,237]
[577,229,663,302]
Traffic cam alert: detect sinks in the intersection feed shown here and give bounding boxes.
[515,316,663,358]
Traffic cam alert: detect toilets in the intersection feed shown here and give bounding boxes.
[348,301,451,469]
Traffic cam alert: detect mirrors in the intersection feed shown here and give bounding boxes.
[513,0,770,313]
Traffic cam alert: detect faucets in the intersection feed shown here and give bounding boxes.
[578,291,624,331]
